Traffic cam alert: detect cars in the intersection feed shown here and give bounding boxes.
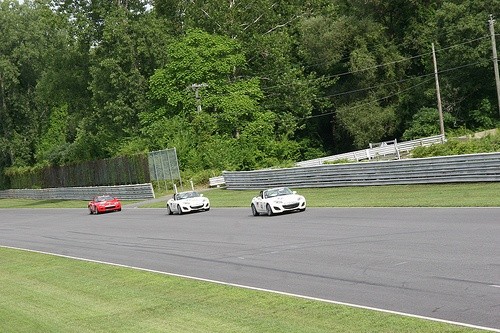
[88,196,122,214]
[165,191,210,214]
[251,186,307,215]
